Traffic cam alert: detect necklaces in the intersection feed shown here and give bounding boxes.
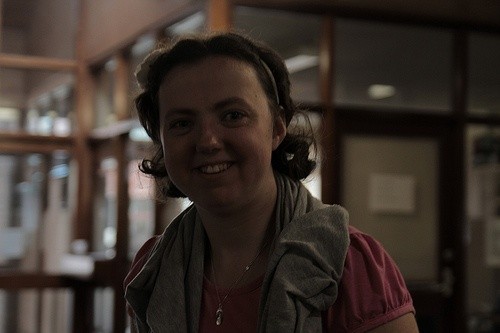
[208,249,263,326]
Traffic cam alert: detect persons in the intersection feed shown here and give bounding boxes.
[118,31,420,333]
